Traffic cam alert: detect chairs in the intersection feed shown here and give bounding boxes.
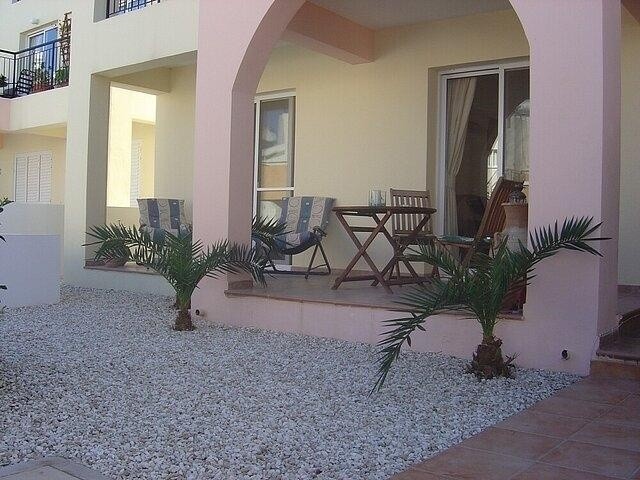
[254,195,335,278]
[136,198,192,247]
[389,176,524,287]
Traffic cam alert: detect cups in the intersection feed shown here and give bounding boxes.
[371,190,382,206]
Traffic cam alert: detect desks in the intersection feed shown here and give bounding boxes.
[331,206,437,298]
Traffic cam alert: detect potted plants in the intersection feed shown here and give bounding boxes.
[32,62,68,92]
